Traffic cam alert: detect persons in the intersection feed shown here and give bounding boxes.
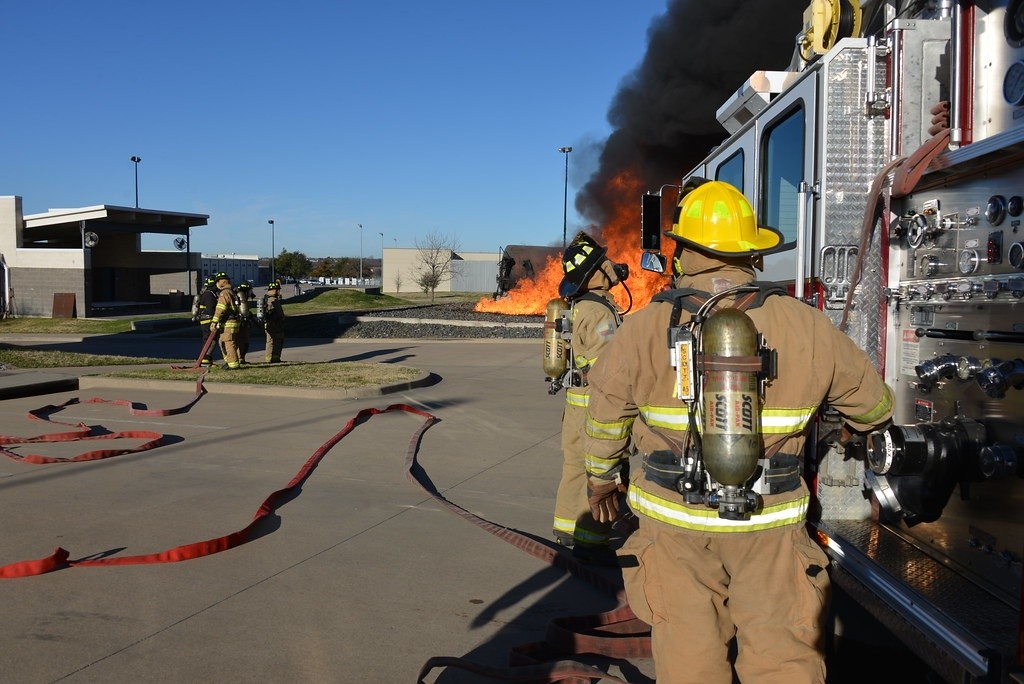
[192,272,256,370]
[262,282,288,363]
[553,181,896,684]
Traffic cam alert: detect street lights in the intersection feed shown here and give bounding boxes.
[129,155,141,208]
[379,232,384,257]
[357,223,362,278]
[267,219,275,282]
[392,238,397,248]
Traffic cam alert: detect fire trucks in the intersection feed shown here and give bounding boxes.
[637,0,1024,684]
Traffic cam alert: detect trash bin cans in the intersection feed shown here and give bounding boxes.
[294,284,301,296]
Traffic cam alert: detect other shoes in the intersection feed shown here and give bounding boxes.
[557,536,575,546]
[221,363,229,370]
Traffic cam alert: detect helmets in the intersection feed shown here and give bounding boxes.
[237,280,253,290]
[214,272,231,281]
[663,180,784,257]
[264,282,282,290]
[204,275,217,287]
[558,241,608,298]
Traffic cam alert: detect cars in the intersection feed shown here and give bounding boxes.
[299,279,308,284]
[307,279,324,286]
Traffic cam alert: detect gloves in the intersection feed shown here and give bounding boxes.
[585,471,626,523]
[841,423,855,442]
